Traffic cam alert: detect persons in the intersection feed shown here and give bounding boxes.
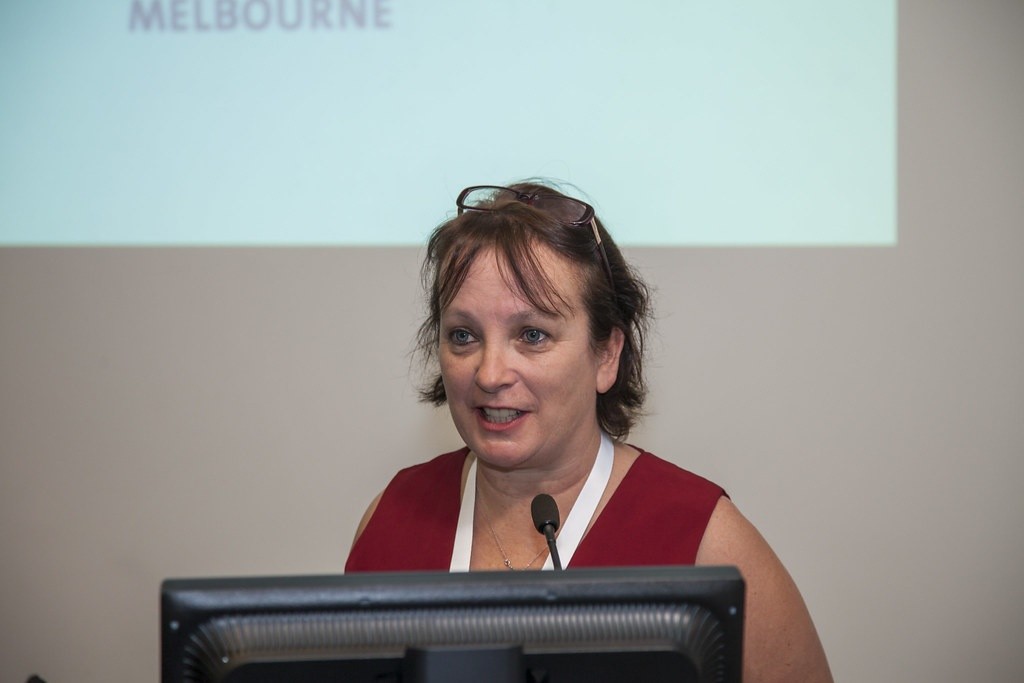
[341,183,833,683]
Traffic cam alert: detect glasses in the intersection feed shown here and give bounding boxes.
[456,186,613,285]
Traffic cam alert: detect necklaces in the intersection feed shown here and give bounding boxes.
[474,502,548,571]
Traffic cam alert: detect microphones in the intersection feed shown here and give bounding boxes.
[530,493,562,572]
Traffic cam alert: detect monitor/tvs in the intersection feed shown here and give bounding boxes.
[162,565,746,683]
